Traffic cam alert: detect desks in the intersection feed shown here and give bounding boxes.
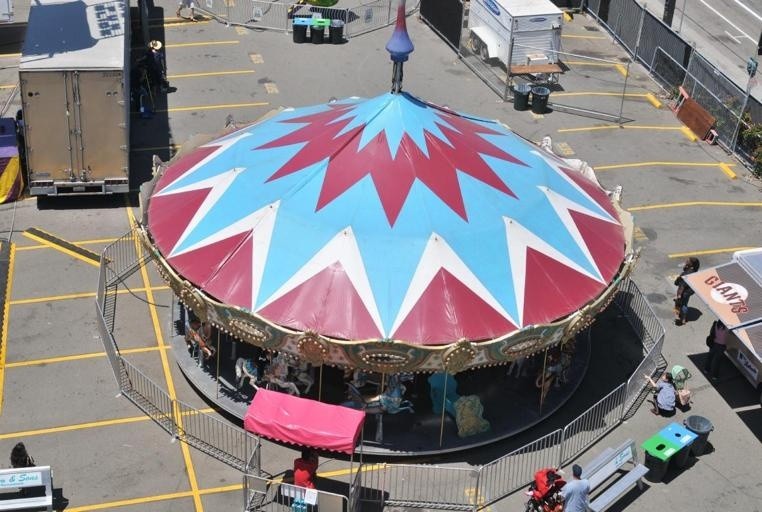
[508,63,563,88]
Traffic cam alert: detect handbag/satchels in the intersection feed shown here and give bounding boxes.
[706,335,715,348]
[675,277,695,295]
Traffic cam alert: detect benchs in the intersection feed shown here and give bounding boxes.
[0,465,54,512]
[581,439,650,512]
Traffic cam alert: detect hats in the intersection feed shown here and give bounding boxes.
[573,464,582,476]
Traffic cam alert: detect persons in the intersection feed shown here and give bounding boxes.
[643,371,677,417]
[703,319,728,382]
[505,358,522,379]
[10,442,37,490]
[295,450,317,512]
[674,257,700,324]
[556,464,590,512]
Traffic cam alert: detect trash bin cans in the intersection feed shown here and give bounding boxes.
[310,18,329,43]
[327,19,344,43]
[291,18,307,43]
[511,83,530,110]
[531,86,551,112]
[642,435,679,484]
[683,413,714,457]
[662,422,700,469]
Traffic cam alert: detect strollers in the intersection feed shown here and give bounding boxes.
[670,366,693,411]
[525,470,567,512]
